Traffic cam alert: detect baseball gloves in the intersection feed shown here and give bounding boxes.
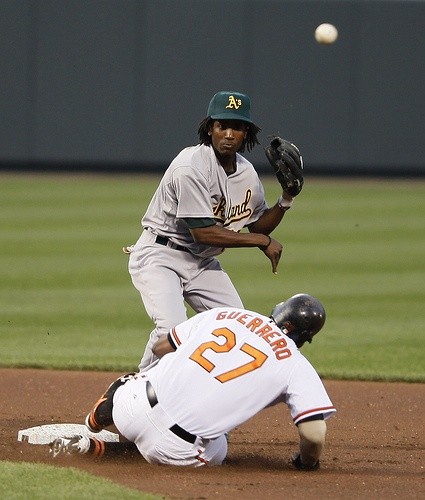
[265,136,304,196]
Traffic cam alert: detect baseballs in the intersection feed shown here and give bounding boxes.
[315,23,338,45]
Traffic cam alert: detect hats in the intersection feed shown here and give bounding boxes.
[206,91,254,124]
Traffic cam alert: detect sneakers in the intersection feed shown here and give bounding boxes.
[85,373,137,433]
[49,435,105,463]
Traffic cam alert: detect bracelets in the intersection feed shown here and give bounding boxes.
[278,195,294,211]
[263,234,271,248]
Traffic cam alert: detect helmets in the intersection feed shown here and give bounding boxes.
[272,294,325,348]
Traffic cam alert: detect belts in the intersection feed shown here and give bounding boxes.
[155,236,188,251]
[146,381,197,444]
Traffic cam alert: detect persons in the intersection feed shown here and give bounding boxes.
[122,89,303,371]
[51,294,336,468]
